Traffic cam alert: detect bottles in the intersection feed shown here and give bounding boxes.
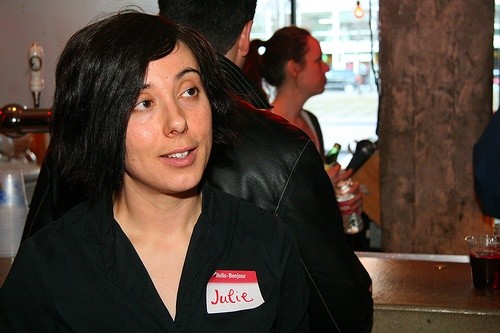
[29,42,44,108]
[335,177,364,233]
[324,143,341,171]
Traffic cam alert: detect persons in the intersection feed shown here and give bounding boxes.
[247,25,371,251]
[156,0,375,333]
[0,10,338,333]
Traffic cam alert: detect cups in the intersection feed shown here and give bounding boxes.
[0,162,30,258]
[465,234,500,297]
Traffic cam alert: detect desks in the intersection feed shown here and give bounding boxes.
[341,251,500,333]
